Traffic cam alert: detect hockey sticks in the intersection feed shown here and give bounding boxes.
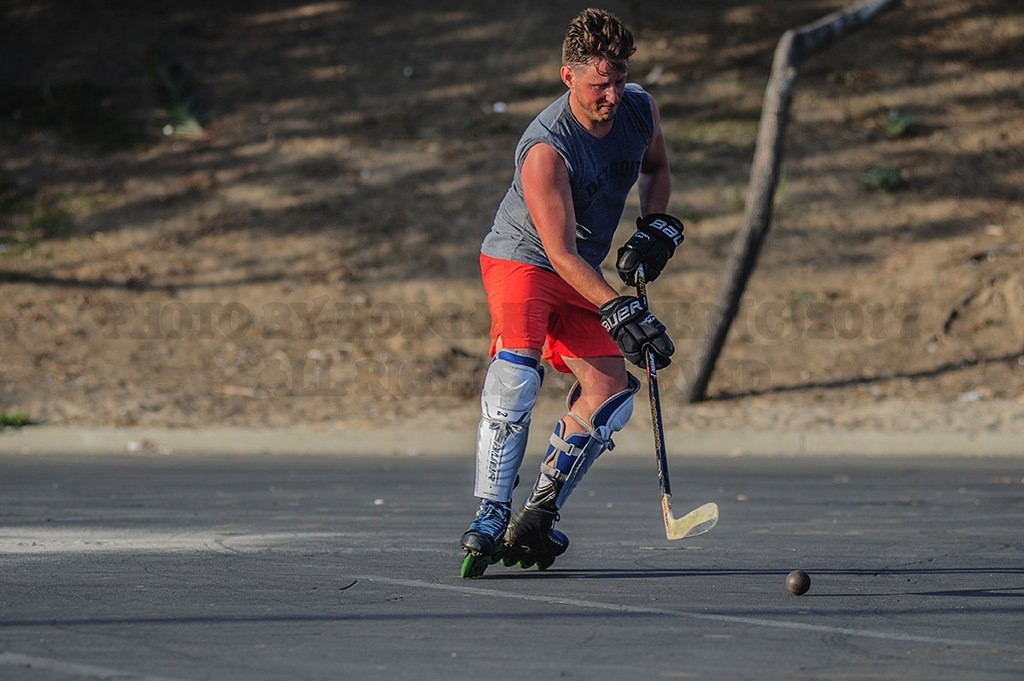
[633,261,720,542]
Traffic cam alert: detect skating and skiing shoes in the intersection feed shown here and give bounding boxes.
[460,474,519,579]
[500,474,570,571]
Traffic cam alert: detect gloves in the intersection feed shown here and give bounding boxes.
[598,295,675,370]
[615,213,685,286]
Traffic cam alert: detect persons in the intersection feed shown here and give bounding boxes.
[459,9,686,581]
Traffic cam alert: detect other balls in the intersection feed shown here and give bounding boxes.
[785,569,812,595]
